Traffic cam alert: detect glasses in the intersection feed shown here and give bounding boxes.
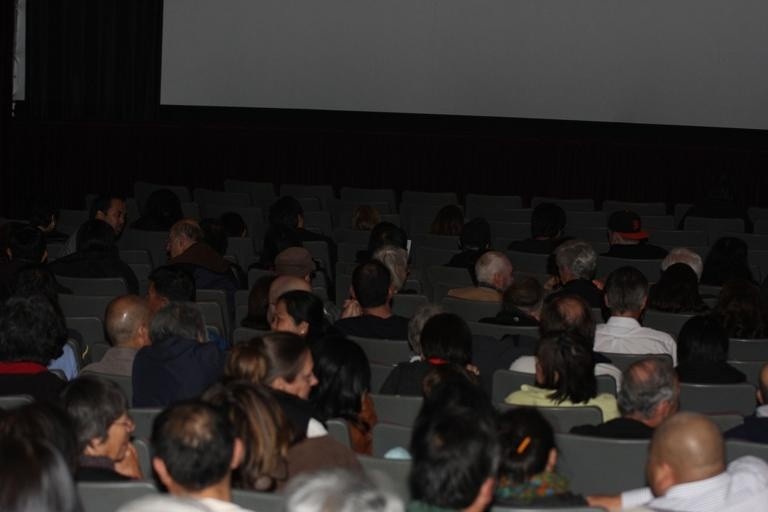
[113,415,135,431]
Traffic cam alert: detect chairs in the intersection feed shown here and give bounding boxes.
[0,178,768,512]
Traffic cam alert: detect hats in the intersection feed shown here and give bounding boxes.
[607,210,649,241]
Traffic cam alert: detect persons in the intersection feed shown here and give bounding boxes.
[0,187,766,511]
